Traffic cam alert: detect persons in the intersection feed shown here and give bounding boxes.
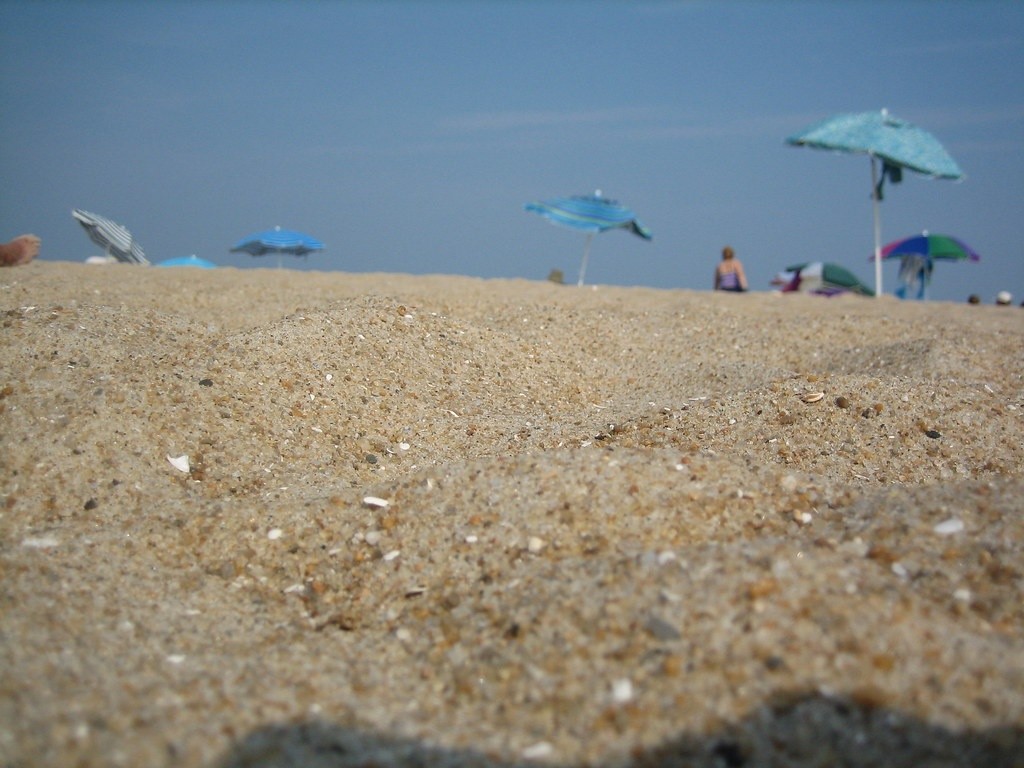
[968,295,980,304]
[0,234,41,267]
[715,246,748,291]
[996,290,1012,306]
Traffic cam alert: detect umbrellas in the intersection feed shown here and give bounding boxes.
[522,187,653,284]
[71,206,151,265]
[230,225,327,267]
[154,254,216,267]
[785,105,965,295]
[868,229,980,260]
[770,261,873,294]
